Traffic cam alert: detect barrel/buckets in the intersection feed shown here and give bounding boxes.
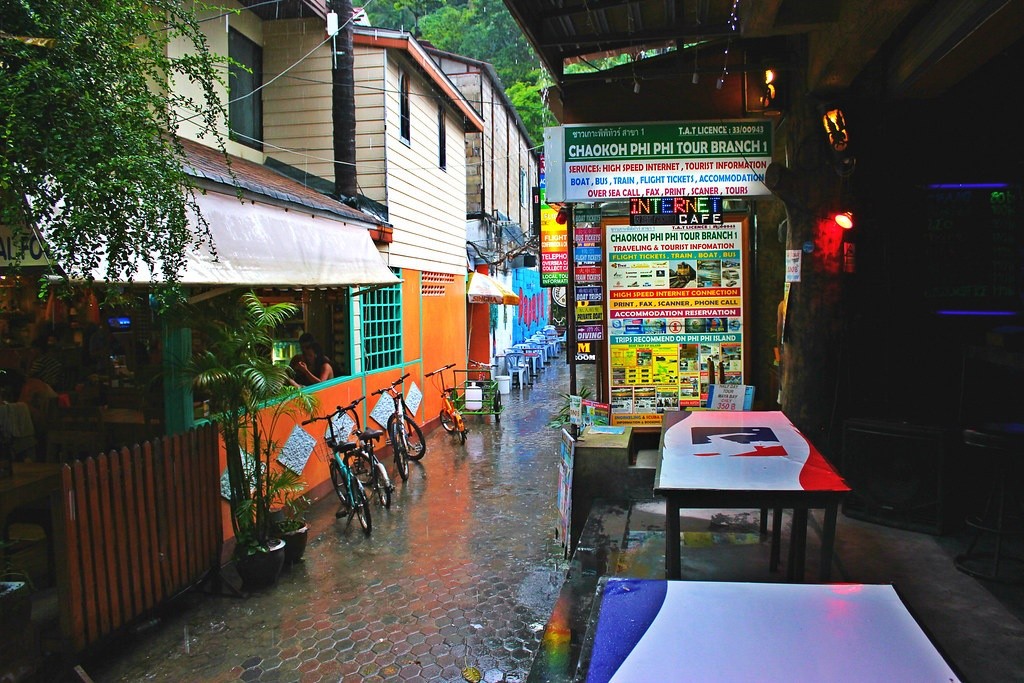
[495,376,511,395]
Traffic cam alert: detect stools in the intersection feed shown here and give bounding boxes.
[953,425,1024,583]
[6,494,57,588]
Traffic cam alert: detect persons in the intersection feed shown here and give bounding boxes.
[296,343,333,383]
[289,333,334,385]
[89,324,114,357]
[28,339,62,384]
[0,368,58,411]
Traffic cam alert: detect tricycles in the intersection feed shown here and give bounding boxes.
[449,360,504,423]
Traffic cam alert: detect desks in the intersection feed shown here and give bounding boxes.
[572,575,970,683]
[0,460,60,582]
[653,409,854,585]
[75,406,163,450]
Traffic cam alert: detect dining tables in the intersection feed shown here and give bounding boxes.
[521,348,545,370]
[496,353,539,380]
[543,334,558,338]
[538,343,550,365]
[541,338,560,359]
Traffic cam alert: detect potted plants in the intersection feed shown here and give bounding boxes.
[178,289,312,587]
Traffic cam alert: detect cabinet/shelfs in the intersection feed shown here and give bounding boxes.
[269,302,309,343]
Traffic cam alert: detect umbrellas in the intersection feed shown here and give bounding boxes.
[466,269,520,358]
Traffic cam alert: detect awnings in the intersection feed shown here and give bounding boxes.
[10,163,405,296]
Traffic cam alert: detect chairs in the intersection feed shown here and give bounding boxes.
[505,352,529,390]
[47,430,96,462]
[504,323,568,383]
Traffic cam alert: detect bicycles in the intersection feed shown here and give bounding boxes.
[552,316,565,328]
[337,386,396,513]
[298,404,376,533]
[428,363,470,444]
[371,370,427,482]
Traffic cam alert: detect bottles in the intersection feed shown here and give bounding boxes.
[465,381,482,410]
